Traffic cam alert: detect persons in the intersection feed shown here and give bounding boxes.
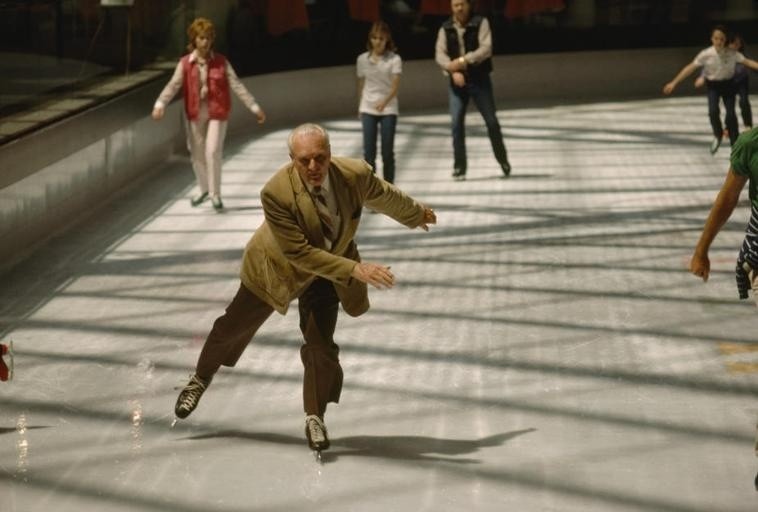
[689,127,757,307]
[173,122,436,450]
[434,2,511,177]
[355,20,403,185]
[663,25,758,153]
[151,18,265,211]
[693,33,754,136]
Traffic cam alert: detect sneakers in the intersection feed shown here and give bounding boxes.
[306,415,330,451]
[710,138,721,153]
[191,191,208,207]
[213,198,222,209]
[453,167,464,176]
[500,161,510,175]
[175,379,207,418]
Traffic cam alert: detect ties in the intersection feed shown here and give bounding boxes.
[315,188,334,251]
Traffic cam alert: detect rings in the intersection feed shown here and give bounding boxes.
[428,207,435,213]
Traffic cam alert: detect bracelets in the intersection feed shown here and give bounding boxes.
[458,55,468,68]
